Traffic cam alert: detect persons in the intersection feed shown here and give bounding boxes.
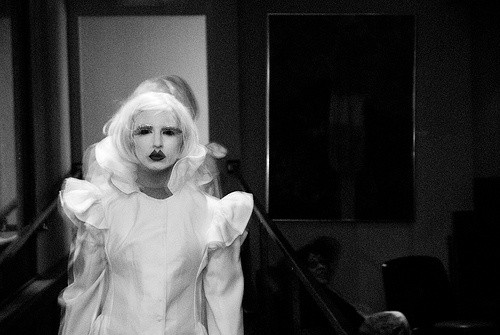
[54,89,254,335]
[298,233,414,335]
[79,74,222,200]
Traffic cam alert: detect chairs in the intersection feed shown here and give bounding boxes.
[382,252,490,335]
